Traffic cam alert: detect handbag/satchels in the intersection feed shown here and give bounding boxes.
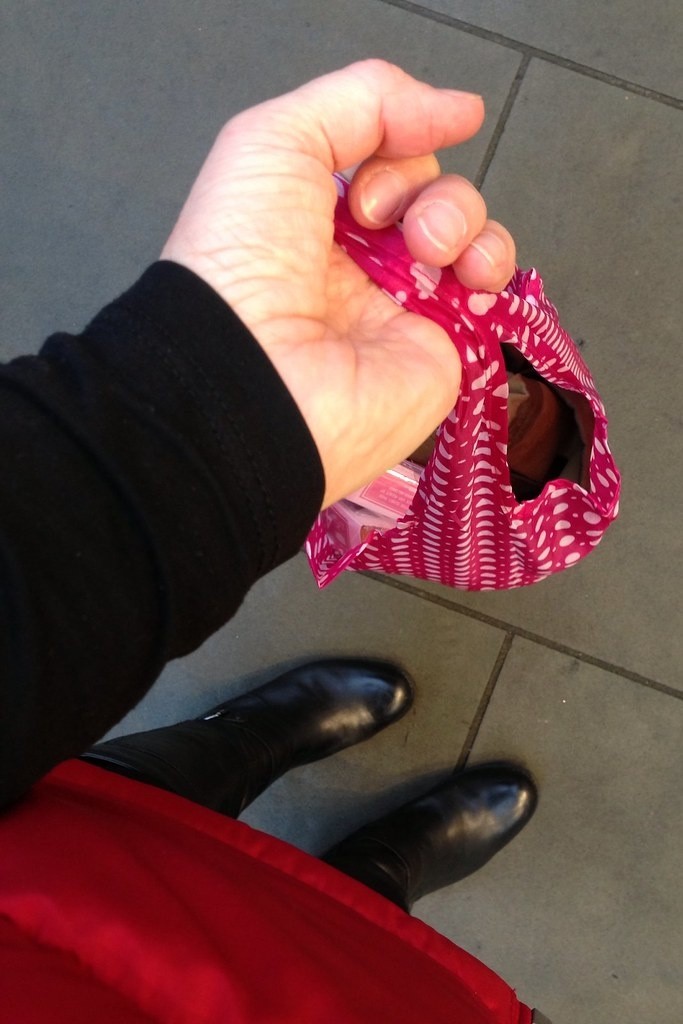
[308,173,622,592]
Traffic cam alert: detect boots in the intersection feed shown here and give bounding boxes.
[316,763,539,914]
[80,657,416,817]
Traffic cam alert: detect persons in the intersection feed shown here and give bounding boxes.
[0,57,555,1024]
